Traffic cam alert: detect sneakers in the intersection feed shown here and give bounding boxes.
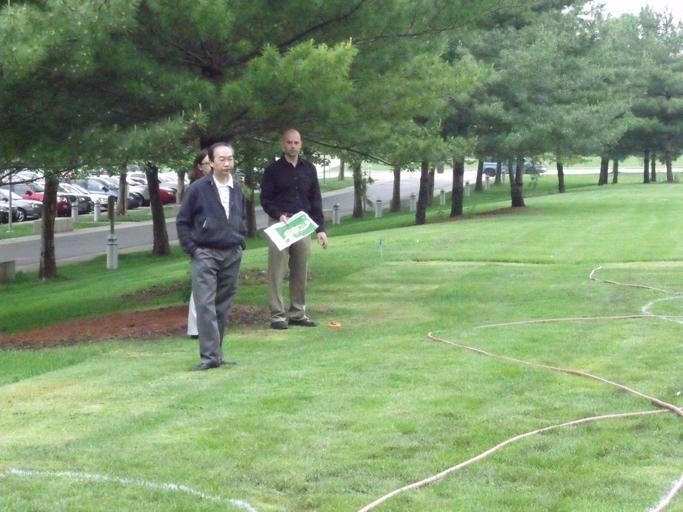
[288,319,317,327]
[192,362,219,371]
[271,320,288,330]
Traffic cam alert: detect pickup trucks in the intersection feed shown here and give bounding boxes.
[482,156,546,177]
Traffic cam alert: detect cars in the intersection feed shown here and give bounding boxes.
[0,165,176,222]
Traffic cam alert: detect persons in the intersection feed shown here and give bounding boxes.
[185,148,213,339]
[176,142,247,371]
[259,127,328,329]
